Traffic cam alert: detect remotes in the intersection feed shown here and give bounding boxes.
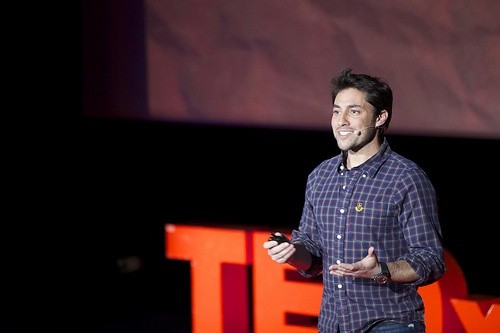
[268,236,290,246]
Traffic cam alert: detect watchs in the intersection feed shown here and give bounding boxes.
[371,262,391,288]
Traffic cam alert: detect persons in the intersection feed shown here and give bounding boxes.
[263,66,447,333]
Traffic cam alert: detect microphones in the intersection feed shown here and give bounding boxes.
[354,126,375,136]
